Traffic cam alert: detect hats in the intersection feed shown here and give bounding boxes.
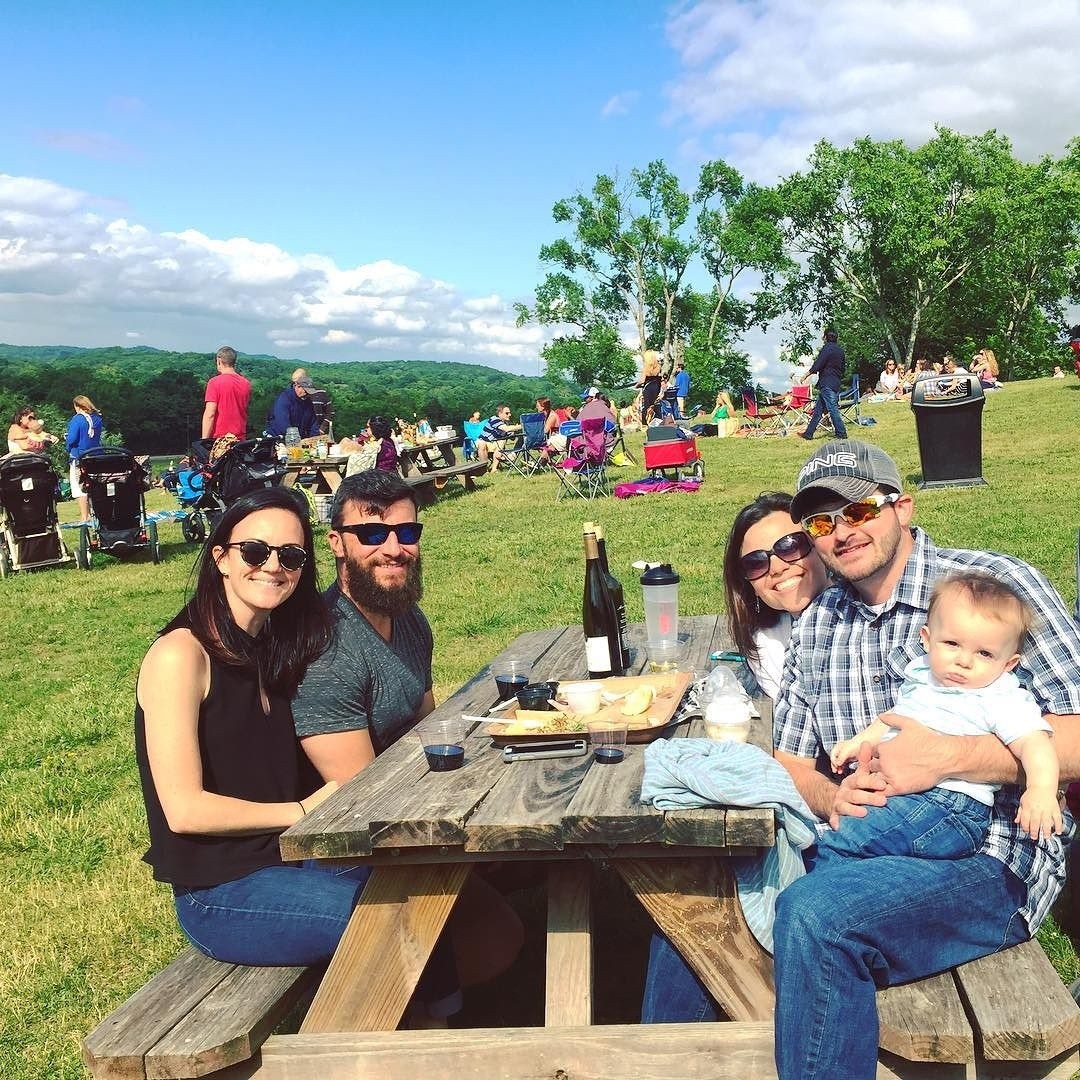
[297,376,316,394]
[790,439,903,524]
[579,387,599,398]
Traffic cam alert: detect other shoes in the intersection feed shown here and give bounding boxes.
[995,381,1004,388]
[797,432,811,440]
[831,434,848,441]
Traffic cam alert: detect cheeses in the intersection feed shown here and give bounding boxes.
[620,688,652,715]
[515,709,566,721]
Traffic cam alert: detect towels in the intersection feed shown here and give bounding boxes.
[636,733,826,956]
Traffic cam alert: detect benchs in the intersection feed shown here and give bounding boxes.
[81,943,310,1079]
[870,937,1080,1080]
[422,461,492,498]
[393,474,437,512]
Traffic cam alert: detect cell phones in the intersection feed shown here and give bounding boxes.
[504,738,586,762]
[711,650,745,662]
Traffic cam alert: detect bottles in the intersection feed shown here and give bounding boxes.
[582,521,624,680]
[594,525,631,669]
[640,564,680,661]
[285,427,303,461]
[490,658,532,702]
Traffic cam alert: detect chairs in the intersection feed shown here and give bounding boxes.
[461,418,492,460]
[673,404,703,423]
[837,373,863,429]
[740,387,787,441]
[780,385,834,437]
[492,413,547,481]
[553,418,625,506]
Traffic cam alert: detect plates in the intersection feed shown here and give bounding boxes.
[483,672,693,747]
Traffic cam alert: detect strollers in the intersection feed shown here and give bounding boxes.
[1,453,80,580]
[182,433,289,542]
[77,446,159,570]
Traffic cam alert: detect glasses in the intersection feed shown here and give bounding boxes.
[335,522,423,545]
[732,531,813,581]
[888,364,894,366]
[25,415,35,422]
[224,542,308,572]
[800,493,904,538]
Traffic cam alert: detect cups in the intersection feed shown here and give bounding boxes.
[702,715,752,744]
[642,639,685,674]
[522,682,560,701]
[413,720,465,771]
[697,693,751,715]
[319,446,327,459]
[587,721,629,764]
[514,689,553,712]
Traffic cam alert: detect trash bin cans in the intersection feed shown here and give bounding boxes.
[911,374,985,481]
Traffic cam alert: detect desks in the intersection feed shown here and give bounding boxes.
[254,612,864,1080]
[286,454,349,496]
[393,436,459,469]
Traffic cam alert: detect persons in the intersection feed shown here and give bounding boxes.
[285,463,549,1026]
[636,438,1080,1079]
[136,486,467,1028]
[6,328,1004,543]
